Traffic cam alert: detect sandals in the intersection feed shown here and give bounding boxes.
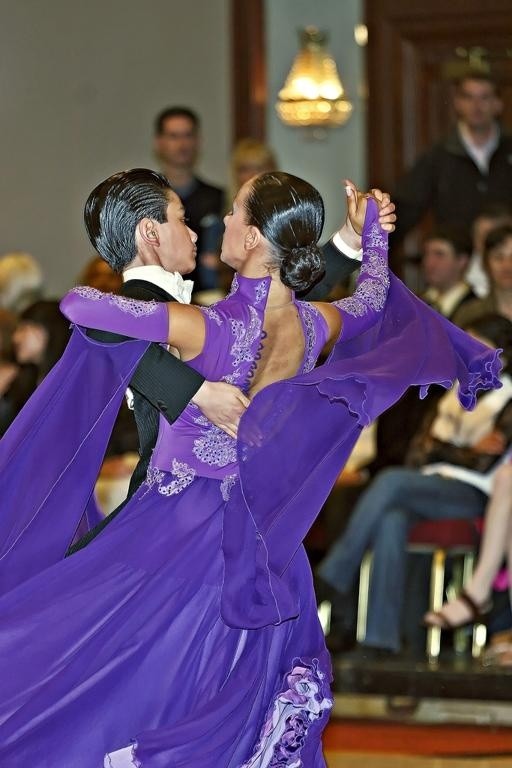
[422,592,497,629]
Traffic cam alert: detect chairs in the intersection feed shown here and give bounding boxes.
[353,514,484,669]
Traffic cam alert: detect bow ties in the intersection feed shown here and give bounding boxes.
[175,272,194,304]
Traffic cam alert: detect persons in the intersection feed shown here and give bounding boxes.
[0,171,503,768]
[65,168,397,560]
[229,137,277,210]
[151,105,225,287]
[3,223,512,668]
[389,71,512,247]
